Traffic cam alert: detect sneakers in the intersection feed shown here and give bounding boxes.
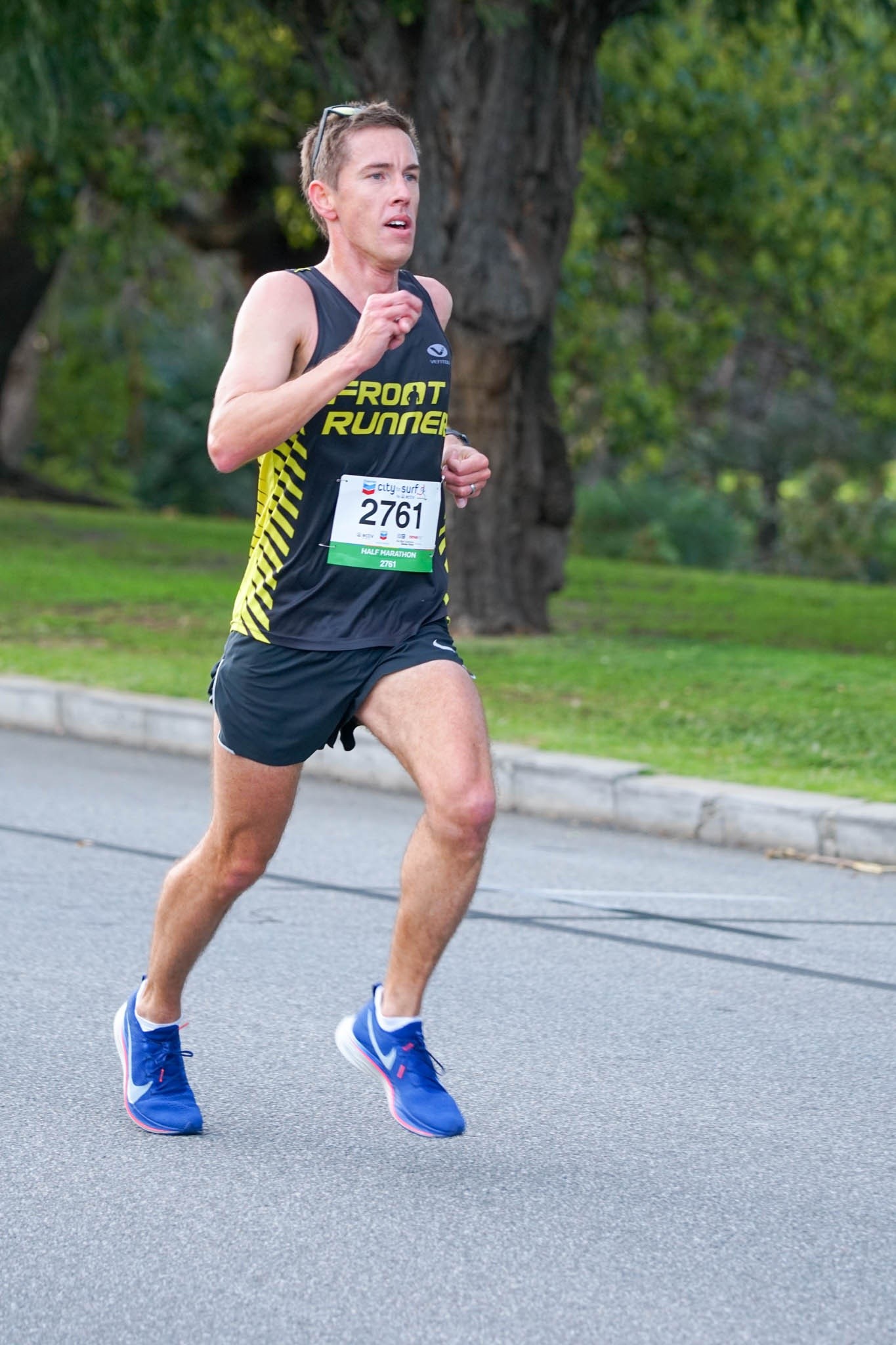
[112,975,206,1136]
[334,981,466,1139]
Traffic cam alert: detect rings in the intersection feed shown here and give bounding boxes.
[470,484,476,496]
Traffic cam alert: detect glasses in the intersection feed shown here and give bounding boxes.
[311,104,365,174]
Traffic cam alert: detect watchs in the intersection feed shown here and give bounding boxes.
[445,429,471,447]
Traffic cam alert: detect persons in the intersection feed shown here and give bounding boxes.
[110,101,496,1135]
